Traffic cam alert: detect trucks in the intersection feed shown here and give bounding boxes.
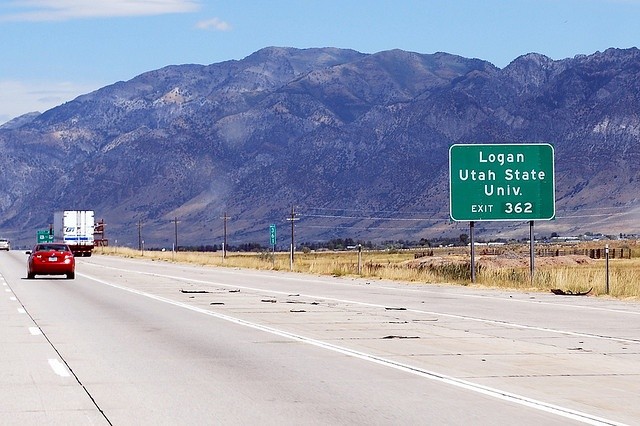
[54,209,95,256]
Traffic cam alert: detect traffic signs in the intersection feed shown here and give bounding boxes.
[448,142,555,221]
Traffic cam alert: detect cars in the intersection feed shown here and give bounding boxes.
[26,242,75,278]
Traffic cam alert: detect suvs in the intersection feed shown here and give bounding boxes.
[0,238,10,251]
